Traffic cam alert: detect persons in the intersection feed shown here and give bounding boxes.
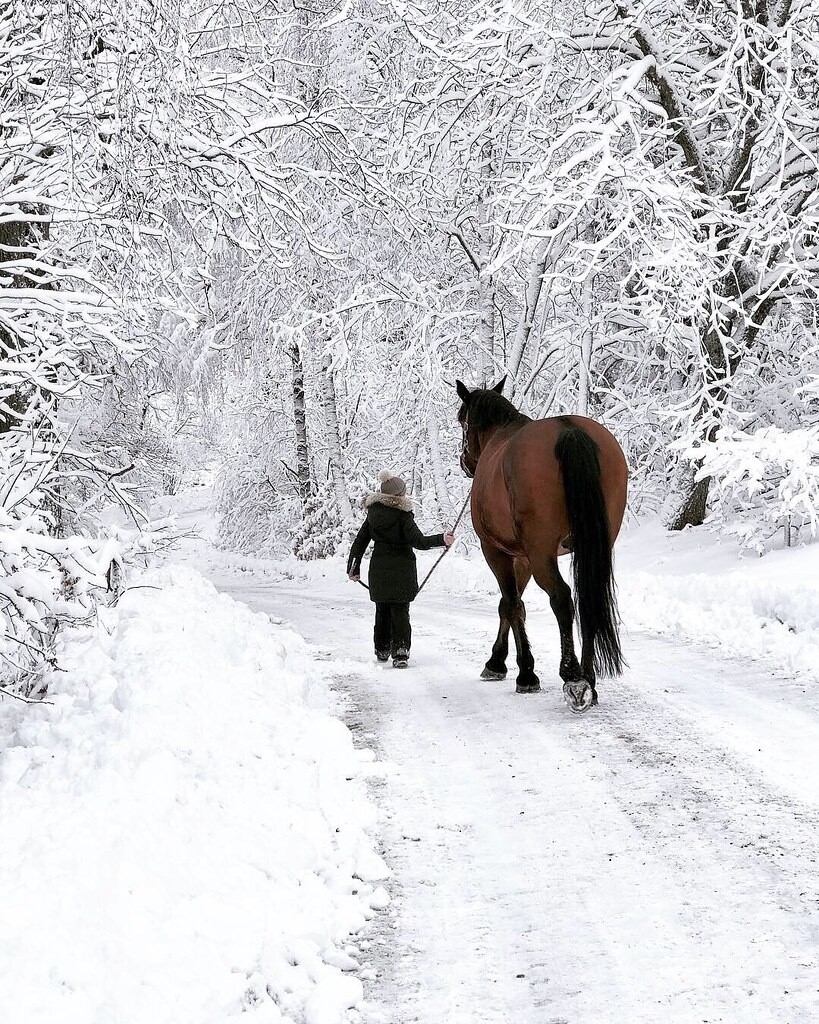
[347,477,456,667]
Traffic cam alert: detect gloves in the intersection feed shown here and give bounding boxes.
[349,574,360,582]
[444,532,455,546]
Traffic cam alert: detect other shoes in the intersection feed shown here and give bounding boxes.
[393,656,408,669]
[377,656,388,663]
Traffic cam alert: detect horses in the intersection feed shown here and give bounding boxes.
[456,375,627,714]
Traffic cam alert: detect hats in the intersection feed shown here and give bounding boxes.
[378,468,406,495]
